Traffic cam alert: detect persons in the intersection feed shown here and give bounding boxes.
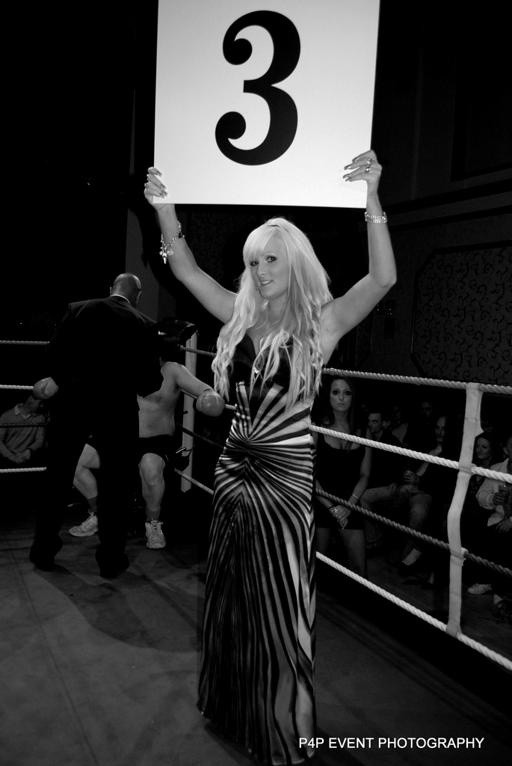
[27,273,164,581]
[33,361,226,550]
[0,393,46,469]
[312,375,512,618]
[143,150,400,765]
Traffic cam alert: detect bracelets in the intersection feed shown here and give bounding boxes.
[160,220,186,247]
[363,211,388,224]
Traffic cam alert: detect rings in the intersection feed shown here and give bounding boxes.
[366,158,372,166]
[364,167,370,173]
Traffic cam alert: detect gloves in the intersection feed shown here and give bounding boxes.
[196,389,226,417]
[32,376,58,399]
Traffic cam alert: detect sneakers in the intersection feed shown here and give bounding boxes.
[467,582,492,595]
[145,519,166,549]
[68,508,98,537]
